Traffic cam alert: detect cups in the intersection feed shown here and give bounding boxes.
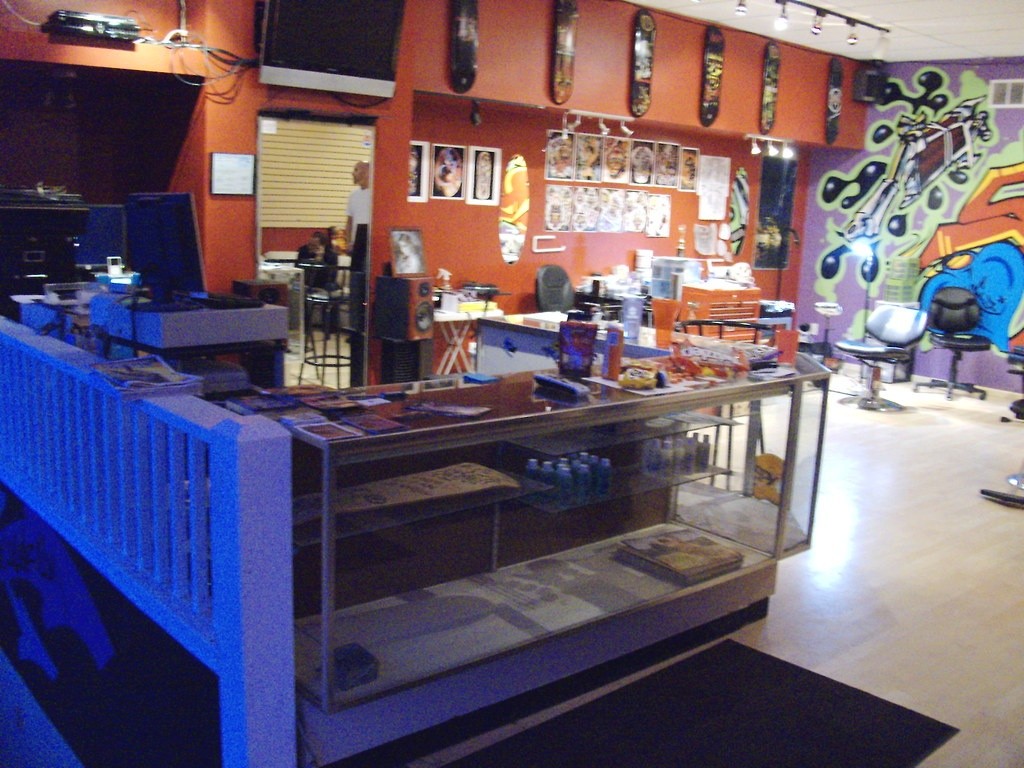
[653,299,680,347]
[622,295,643,338]
[775,329,797,368]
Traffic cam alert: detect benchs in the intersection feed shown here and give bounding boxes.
[269,250,350,331]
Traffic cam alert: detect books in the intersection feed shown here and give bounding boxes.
[609,522,744,587]
[90,352,206,399]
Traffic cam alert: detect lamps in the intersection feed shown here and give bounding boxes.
[773,0,789,32]
[734,0,749,18]
[565,113,586,135]
[809,11,829,36]
[845,22,862,44]
[619,119,636,137]
[596,117,612,136]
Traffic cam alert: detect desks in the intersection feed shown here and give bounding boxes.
[434,306,505,375]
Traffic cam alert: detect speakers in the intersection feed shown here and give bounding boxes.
[232,280,288,349]
[375,275,433,340]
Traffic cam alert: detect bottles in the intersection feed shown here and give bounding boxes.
[525,452,611,510]
[601,328,623,381]
[441,289,497,313]
[642,432,710,484]
[563,272,612,321]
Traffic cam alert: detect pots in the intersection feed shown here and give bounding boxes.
[462,281,510,296]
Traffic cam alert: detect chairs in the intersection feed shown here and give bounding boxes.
[834,299,928,414]
[533,265,580,315]
[1002,346,1024,421]
[672,318,780,490]
[911,274,994,402]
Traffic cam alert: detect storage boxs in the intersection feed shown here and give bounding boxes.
[650,255,701,279]
[650,277,674,296]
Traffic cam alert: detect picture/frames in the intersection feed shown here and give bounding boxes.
[431,142,467,202]
[465,143,503,207]
[405,138,432,203]
[386,225,430,278]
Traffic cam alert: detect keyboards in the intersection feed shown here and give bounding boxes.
[145,289,266,309]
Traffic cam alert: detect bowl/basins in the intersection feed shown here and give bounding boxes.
[612,248,653,279]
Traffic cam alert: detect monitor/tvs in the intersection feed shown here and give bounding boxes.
[258,0,404,99]
[127,192,207,312]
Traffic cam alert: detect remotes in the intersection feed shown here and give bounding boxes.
[535,373,590,398]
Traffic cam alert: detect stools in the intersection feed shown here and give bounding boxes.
[292,259,358,386]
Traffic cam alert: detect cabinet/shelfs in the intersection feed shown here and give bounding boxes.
[502,408,744,520]
[651,285,762,339]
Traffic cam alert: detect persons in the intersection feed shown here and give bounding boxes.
[343,160,371,344]
[294,230,339,332]
